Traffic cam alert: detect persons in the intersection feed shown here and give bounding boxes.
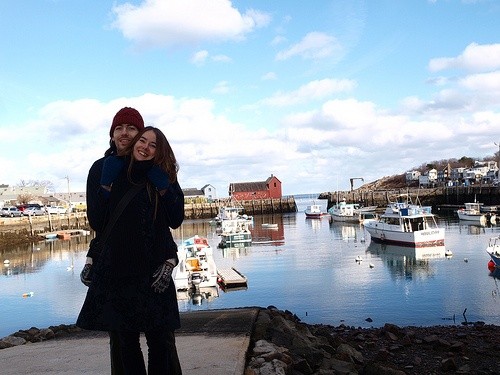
[86,106,183,231]
[73,126,185,375]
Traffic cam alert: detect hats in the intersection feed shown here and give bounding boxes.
[110,106,145,137]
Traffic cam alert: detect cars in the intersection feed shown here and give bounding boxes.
[0,204,68,218]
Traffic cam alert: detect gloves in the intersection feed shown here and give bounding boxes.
[100,155,122,186]
[147,165,170,193]
[151,261,175,296]
[79,265,94,288]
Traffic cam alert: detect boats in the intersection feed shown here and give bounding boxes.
[363,203,446,250]
[261,223,278,228]
[457,202,486,221]
[486,244,500,268]
[209,211,253,247]
[171,235,218,289]
[328,203,360,221]
[304,204,323,219]
